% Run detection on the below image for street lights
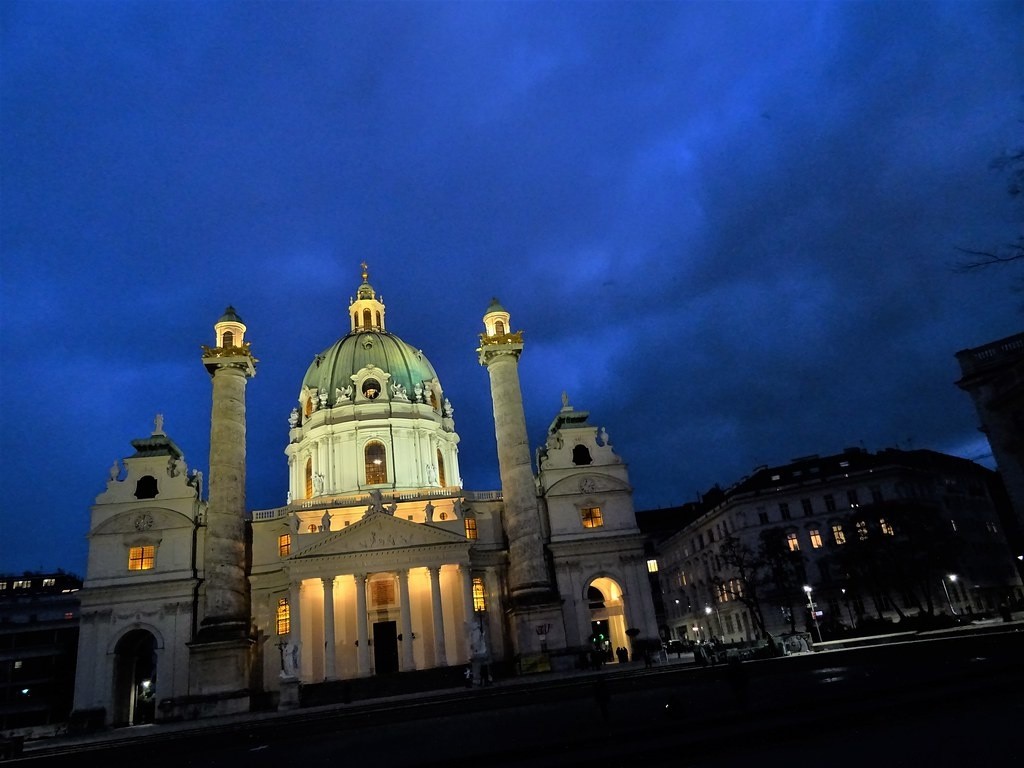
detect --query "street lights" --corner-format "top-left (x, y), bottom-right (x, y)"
top-left (940, 570), bottom-right (957, 615)
top-left (804, 586), bottom-right (822, 641)
top-left (705, 600), bottom-right (725, 644)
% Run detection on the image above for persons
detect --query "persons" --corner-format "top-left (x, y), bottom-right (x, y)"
top-left (471, 621), bottom-right (483, 654)
top-left (590, 646), bottom-right (628, 672)
top-left (322, 510), bottom-right (334, 532)
top-left (369, 488), bottom-right (386, 513)
top-left (290, 510), bottom-right (304, 535)
top-left (280, 632), bottom-right (296, 676)
top-left (453, 496), bottom-right (466, 520)
top-left (422, 501), bottom-right (436, 523)
